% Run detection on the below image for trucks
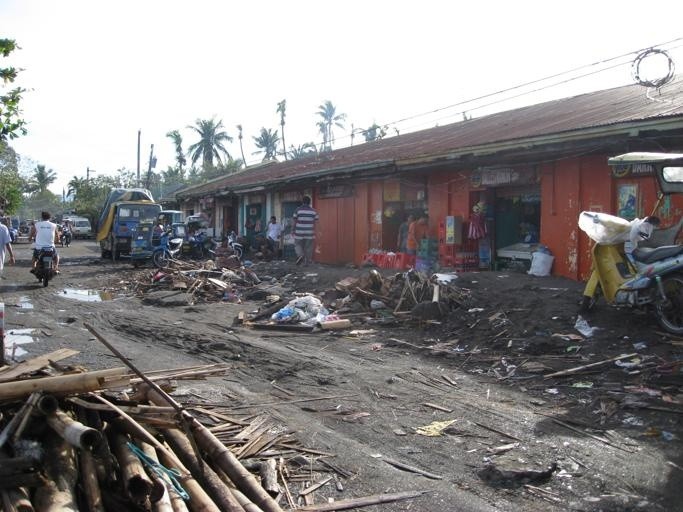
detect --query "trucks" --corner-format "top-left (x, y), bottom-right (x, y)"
top-left (62, 214), bottom-right (91, 239)
top-left (96, 188), bottom-right (162, 261)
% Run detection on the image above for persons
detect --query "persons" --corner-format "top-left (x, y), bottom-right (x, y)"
top-left (29, 211), bottom-right (73, 275)
top-left (291, 196), bottom-right (319, 268)
top-left (0, 216), bottom-right (15, 270)
top-left (397, 212), bottom-right (431, 256)
top-left (266, 216), bottom-right (281, 258)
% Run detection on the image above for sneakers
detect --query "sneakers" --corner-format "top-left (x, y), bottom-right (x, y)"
top-left (295, 256), bottom-right (303, 265)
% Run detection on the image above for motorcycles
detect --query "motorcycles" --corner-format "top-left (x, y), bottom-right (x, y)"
top-left (583, 213), bottom-right (683, 335)
top-left (129, 210), bottom-right (243, 268)
top-left (30, 232), bottom-right (71, 286)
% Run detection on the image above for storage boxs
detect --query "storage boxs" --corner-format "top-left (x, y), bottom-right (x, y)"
top-left (415, 239), bottom-right (438, 274)
